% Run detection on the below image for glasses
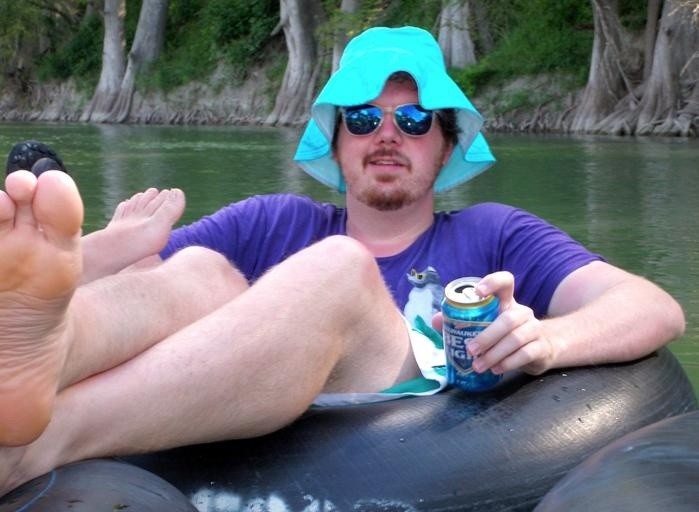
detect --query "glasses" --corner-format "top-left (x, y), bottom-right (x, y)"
top-left (336, 103), bottom-right (444, 137)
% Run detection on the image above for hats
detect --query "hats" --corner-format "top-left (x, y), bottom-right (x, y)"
top-left (293, 26), bottom-right (497, 193)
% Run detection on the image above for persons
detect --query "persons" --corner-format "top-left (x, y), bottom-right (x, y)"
top-left (0, 26), bottom-right (687, 492)
top-left (78, 185), bottom-right (185, 286)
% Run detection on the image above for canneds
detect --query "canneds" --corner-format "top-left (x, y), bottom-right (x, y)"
top-left (442, 277), bottom-right (504, 391)
